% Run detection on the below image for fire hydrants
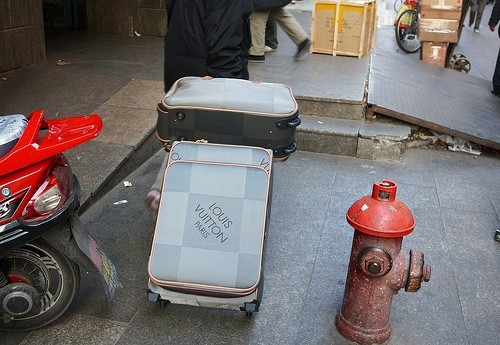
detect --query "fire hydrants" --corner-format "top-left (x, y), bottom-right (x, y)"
top-left (334, 179), bottom-right (433, 345)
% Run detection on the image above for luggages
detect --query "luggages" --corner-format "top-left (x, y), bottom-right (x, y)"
top-left (154, 75), bottom-right (301, 162)
top-left (147, 140), bottom-right (274, 317)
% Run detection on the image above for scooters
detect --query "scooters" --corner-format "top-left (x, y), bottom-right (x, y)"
top-left (0, 108), bottom-right (119, 333)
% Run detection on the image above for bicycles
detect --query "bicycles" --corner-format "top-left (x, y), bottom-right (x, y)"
top-left (394, 0), bottom-right (422, 54)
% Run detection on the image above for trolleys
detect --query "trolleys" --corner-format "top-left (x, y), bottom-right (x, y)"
top-left (419, 0), bottom-right (472, 74)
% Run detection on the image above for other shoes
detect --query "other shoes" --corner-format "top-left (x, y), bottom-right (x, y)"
top-left (474, 30), bottom-right (479, 33)
top-left (468, 23), bottom-right (471, 28)
top-left (264, 45), bottom-right (278, 53)
top-left (295, 39), bottom-right (311, 58)
top-left (248, 54), bottom-right (264, 62)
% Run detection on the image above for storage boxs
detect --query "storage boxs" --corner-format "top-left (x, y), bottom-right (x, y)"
top-left (422, 41), bottom-right (449, 68)
top-left (417, 14), bottom-right (459, 43)
top-left (310, 0), bottom-right (377, 60)
top-left (420, 0), bottom-right (462, 20)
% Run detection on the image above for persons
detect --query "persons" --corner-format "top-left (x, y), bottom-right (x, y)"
top-left (248, 0), bottom-right (312, 63)
top-left (145, 0), bottom-right (252, 220)
top-left (469, 0), bottom-right (489, 34)
top-left (488, 0), bottom-right (500, 96)
